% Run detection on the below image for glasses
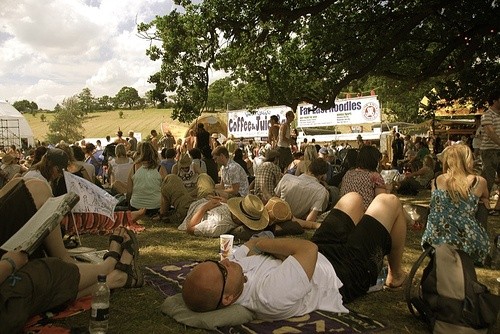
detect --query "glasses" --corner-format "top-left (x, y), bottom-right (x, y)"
top-left (195, 259), bottom-right (228, 310)
top-left (55, 167), bottom-right (63, 173)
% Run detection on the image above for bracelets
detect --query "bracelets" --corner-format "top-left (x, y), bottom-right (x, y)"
top-left (2, 258), bottom-right (16, 277)
top-left (254, 238), bottom-right (265, 253)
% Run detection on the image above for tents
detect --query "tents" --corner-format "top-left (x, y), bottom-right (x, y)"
top-left (0, 99), bottom-right (36, 149)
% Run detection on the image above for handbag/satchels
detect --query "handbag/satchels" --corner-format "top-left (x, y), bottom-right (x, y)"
top-left (229, 225), bottom-right (262, 241)
top-left (272, 221), bottom-right (304, 236)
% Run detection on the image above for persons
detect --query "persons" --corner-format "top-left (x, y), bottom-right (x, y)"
top-left (182, 192), bottom-right (408, 315)
top-left (422, 140), bottom-right (491, 263)
top-left (0, 91), bottom-right (500, 233)
top-left (0, 177), bottom-right (82, 264)
top-left (0, 224), bottom-right (144, 334)
top-left (153, 172), bottom-right (270, 238)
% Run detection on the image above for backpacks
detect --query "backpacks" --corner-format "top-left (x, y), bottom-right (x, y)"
top-left (407, 241), bottom-right (498, 330)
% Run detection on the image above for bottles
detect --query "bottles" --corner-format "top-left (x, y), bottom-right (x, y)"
top-left (88, 274), bottom-right (109, 334)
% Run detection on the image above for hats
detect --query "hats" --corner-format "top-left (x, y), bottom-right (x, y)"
top-left (45, 148), bottom-right (68, 172)
top-left (115, 131), bottom-right (123, 136)
top-left (265, 196), bottom-right (293, 226)
top-left (226, 194), bottom-right (269, 230)
top-left (356, 135), bottom-right (362, 138)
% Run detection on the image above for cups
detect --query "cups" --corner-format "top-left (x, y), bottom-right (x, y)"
top-left (220, 235), bottom-right (235, 260)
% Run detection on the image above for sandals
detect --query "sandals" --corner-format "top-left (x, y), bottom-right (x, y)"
top-left (103, 226), bottom-right (128, 261)
top-left (114, 230), bottom-right (143, 288)
top-left (152, 210), bottom-right (170, 223)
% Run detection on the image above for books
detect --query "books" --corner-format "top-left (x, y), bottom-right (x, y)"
top-left (1, 193), bottom-right (80, 255)
